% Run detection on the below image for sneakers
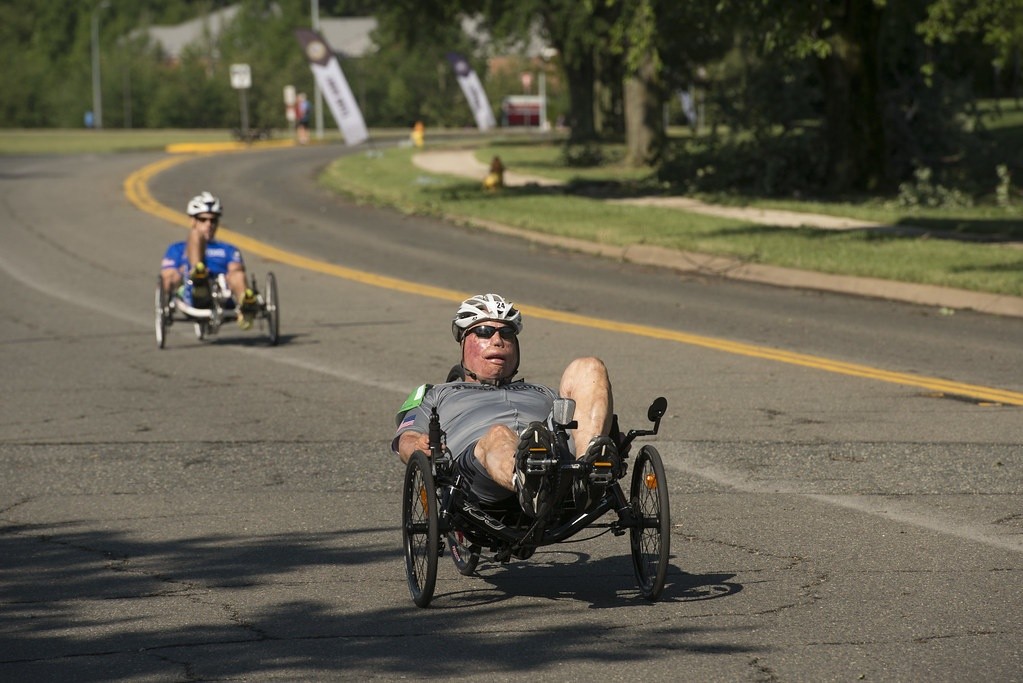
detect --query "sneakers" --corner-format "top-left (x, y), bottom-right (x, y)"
top-left (571, 435), bottom-right (619, 513)
top-left (511, 422), bottom-right (553, 519)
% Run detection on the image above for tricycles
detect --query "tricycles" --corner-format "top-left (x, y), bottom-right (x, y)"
top-left (153, 258), bottom-right (281, 350)
top-left (398, 362), bottom-right (673, 610)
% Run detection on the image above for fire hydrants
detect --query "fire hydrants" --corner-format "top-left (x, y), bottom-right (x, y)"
top-left (481, 154), bottom-right (509, 188)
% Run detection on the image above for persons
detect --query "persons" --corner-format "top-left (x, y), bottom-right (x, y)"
top-left (297, 93), bottom-right (311, 145)
top-left (391, 293), bottom-right (620, 520)
top-left (160, 191), bottom-right (256, 329)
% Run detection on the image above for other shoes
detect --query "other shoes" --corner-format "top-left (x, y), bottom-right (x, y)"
top-left (237, 289), bottom-right (257, 331)
top-left (190, 263), bottom-right (209, 308)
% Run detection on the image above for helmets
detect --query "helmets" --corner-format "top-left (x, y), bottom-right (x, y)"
top-left (187, 191), bottom-right (224, 217)
top-left (451, 293), bottom-right (522, 342)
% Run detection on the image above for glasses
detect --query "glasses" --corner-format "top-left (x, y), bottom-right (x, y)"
top-left (464, 326), bottom-right (517, 340)
top-left (194, 216), bottom-right (218, 224)
